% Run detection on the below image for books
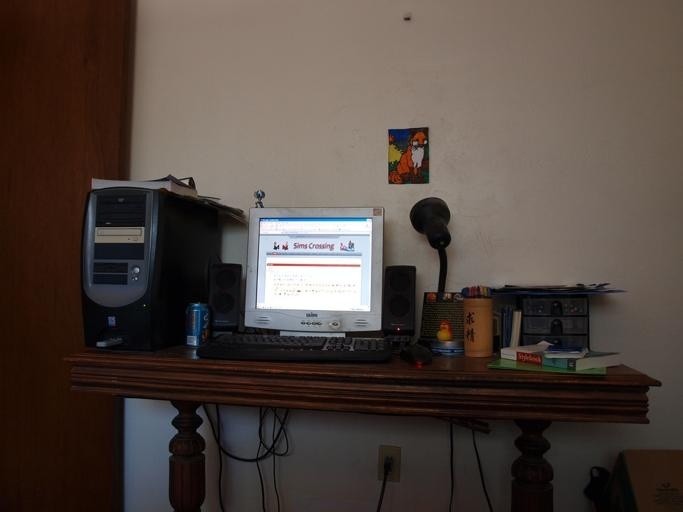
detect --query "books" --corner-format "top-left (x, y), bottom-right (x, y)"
top-left (88, 173), bottom-right (198, 200)
top-left (542, 344), bottom-right (587, 359)
top-left (509, 308), bottom-right (521, 348)
top-left (499, 306), bottom-right (511, 347)
top-left (499, 340), bottom-right (624, 371)
top-left (487, 357), bottom-right (607, 376)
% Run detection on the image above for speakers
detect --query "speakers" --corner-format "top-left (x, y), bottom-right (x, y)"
top-left (206, 262), bottom-right (242, 328)
top-left (385, 264), bottom-right (416, 333)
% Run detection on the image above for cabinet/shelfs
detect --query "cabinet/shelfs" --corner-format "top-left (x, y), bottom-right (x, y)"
top-left (513, 294), bottom-right (590, 352)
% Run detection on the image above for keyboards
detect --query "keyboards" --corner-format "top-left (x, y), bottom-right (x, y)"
top-left (211, 332), bottom-right (391, 364)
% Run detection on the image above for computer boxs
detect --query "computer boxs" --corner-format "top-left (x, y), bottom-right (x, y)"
top-left (80, 189), bottom-right (222, 353)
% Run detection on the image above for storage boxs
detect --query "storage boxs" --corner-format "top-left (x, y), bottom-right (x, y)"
top-left (613, 448), bottom-right (683, 512)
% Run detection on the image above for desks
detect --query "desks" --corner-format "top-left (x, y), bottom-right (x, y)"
top-left (55, 351), bottom-right (663, 512)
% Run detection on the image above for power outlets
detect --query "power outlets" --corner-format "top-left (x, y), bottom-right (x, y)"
top-left (378, 446), bottom-right (402, 480)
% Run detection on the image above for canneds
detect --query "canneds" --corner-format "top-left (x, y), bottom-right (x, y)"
top-left (185, 302), bottom-right (211, 349)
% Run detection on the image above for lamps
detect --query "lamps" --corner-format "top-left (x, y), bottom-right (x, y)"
top-left (410, 195), bottom-right (452, 303)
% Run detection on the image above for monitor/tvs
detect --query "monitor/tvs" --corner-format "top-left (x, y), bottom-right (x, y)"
top-left (243, 206), bottom-right (386, 338)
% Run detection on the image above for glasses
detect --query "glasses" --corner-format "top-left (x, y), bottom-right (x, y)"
top-left (178, 177), bottom-right (197, 190)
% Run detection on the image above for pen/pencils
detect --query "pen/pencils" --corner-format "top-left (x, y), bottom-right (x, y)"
top-left (462, 286), bottom-right (491, 298)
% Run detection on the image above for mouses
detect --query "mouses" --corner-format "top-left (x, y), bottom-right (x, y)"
top-left (403, 343), bottom-right (432, 367)
